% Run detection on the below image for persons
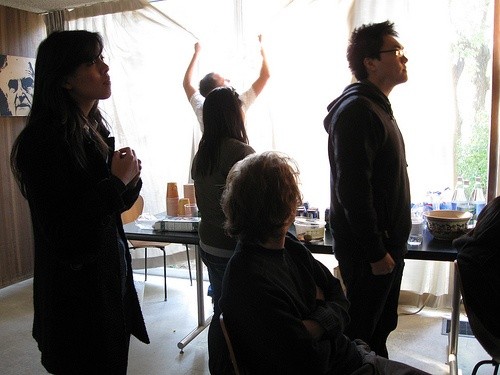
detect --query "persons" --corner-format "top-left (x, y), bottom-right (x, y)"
top-left (218, 152), bottom-right (435, 375)
top-left (182, 34), bottom-right (275, 137)
top-left (192, 86), bottom-right (258, 375)
top-left (324, 21), bottom-right (412, 358)
top-left (453, 195), bottom-right (500, 339)
top-left (0, 55), bottom-right (36, 116)
top-left (11, 30), bottom-right (142, 375)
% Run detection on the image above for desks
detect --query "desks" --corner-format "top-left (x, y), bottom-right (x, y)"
top-left (123, 215), bottom-right (484, 375)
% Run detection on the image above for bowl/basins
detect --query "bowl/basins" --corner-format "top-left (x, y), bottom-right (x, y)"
top-left (423, 210), bottom-right (473, 240)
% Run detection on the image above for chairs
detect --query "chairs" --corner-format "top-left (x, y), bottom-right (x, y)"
top-left (457, 253), bottom-right (500, 375)
top-left (121, 195), bottom-right (192, 301)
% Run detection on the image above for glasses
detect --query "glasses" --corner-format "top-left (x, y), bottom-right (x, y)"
top-left (378, 47), bottom-right (404, 56)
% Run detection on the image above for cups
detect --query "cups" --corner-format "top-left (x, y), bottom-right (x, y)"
top-left (407, 219), bottom-right (424, 245)
top-left (166, 183), bottom-right (198, 220)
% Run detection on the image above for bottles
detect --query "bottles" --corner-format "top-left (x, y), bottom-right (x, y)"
top-left (451, 178), bottom-right (486, 228)
